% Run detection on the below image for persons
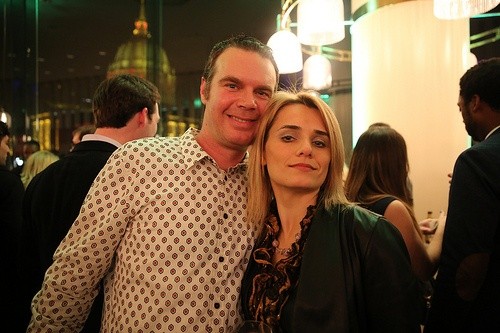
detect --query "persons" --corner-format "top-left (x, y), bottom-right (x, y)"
top-left (422, 60), bottom-right (500, 333)
top-left (235, 77), bottom-right (420, 333)
top-left (343, 122), bottom-right (453, 284)
top-left (24, 74), bottom-right (161, 333)
top-left (0, 119), bottom-right (94, 333)
top-left (26, 33), bottom-right (279, 333)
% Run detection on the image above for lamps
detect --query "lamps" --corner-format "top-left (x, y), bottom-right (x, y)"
top-left (266, 0), bottom-right (345, 91)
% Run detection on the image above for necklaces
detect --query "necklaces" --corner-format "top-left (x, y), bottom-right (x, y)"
top-left (272, 232), bottom-right (301, 257)
